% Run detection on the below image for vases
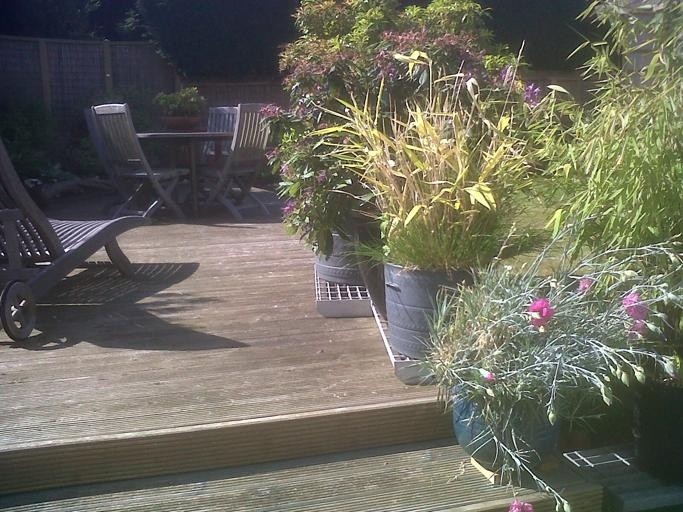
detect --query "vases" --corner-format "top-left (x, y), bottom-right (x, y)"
top-left (446, 346), bottom-right (567, 472)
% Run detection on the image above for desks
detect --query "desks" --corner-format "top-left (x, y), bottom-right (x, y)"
top-left (134, 128), bottom-right (234, 210)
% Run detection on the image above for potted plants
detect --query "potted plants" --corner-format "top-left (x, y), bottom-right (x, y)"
top-left (298, 51), bottom-right (579, 365)
top-left (627, 249), bottom-right (683, 480)
top-left (152, 83), bottom-right (208, 133)
top-left (257, 0), bottom-right (545, 285)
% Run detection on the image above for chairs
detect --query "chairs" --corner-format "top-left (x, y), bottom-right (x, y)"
top-left (0, 126), bottom-right (154, 344)
top-left (89, 100), bottom-right (190, 221)
top-left (188, 98), bottom-right (277, 226)
top-left (196, 100), bottom-right (242, 205)
top-left (78, 104), bottom-right (163, 209)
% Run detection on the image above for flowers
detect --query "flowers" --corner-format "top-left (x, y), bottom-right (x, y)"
top-left (402, 215), bottom-right (683, 511)
